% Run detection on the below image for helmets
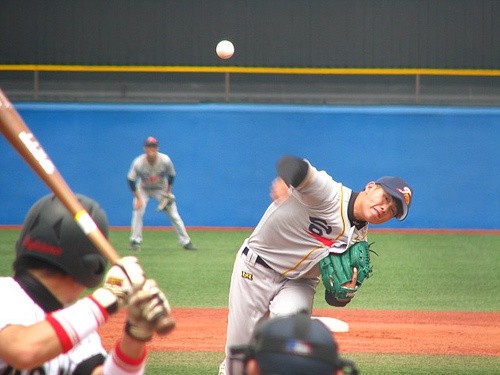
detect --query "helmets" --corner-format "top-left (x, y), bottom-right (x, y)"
top-left (13, 191), bottom-right (109, 288)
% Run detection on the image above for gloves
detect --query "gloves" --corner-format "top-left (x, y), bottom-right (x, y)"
top-left (91, 256), bottom-right (145, 317)
top-left (125, 279), bottom-right (170, 341)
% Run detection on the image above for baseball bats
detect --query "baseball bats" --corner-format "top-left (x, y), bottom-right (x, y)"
top-left (0, 87), bottom-right (175, 335)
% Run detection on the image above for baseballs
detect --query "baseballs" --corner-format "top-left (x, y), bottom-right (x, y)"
top-left (216, 39), bottom-right (235, 60)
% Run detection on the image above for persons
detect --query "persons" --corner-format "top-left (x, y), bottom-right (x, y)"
top-left (127, 137), bottom-right (197, 249)
top-left (229, 313), bottom-right (358, 375)
top-left (217, 152), bottom-right (413, 375)
top-left (0, 188), bottom-right (170, 375)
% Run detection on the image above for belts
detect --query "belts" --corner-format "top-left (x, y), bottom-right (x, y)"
top-left (243, 247), bottom-right (272, 269)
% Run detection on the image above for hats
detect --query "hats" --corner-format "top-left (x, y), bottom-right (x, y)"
top-left (144, 136), bottom-right (158, 146)
top-left (375, 176), bottom-right (414, 221)
top-left (243, 309), bottom-right (337, 366)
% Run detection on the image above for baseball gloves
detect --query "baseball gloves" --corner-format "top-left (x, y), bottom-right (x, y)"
top-left (319, 241), bottom-right (371, 298)
top-left (157, 194), bottom-right (175, 211)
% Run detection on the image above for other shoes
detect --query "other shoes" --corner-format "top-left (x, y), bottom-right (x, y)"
top-left (129, 243), bottom-right (140, 250)
top-left (184, 242), bottom-right (198, 250)
